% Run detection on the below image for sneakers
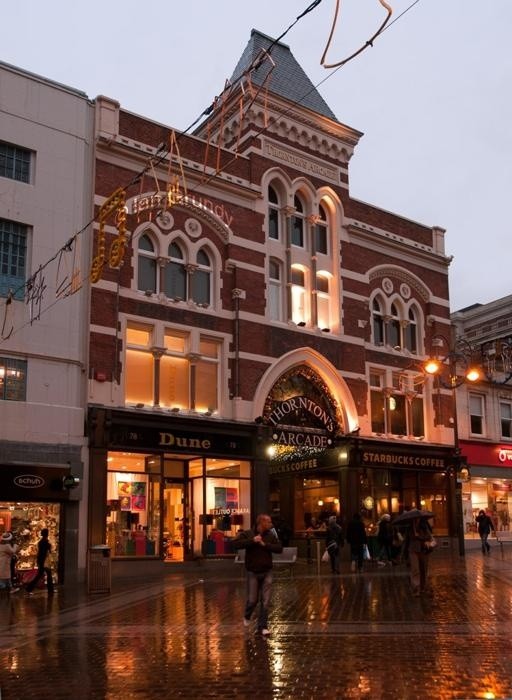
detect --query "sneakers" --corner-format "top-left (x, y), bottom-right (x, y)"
top-left (260, 628), bottom-right (270, 635)
top-left (243, 616), bottom-right (250, 627)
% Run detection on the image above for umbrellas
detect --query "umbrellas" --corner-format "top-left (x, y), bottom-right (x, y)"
top-left (392, 507), bottom-right (436, 525)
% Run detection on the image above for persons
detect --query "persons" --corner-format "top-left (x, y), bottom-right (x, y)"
top-left (474, 509), bottom-right (495, 554)
top-left (119, 496), bottom-right (129, 508)
top-left (0, 528), bottom-right (22, 594)
top-left (23, 528), bottom-right (59, 594)
top-left (226, 514), bottom-right (282, 637)
top-left (324, 510), bottom-right (437, 597)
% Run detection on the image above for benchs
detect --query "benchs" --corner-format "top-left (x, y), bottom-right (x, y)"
top-left (234, 547), bottom-right (297, 582)
top-left (492, 530), bottom-right (512, 552)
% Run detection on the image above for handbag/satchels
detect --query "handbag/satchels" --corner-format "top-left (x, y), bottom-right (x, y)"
top-left (422, 540), bottom-right (436, 555)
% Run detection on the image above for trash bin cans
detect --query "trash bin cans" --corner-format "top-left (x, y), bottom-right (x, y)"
top-left (87, 546), bottom-right (113, 596)
